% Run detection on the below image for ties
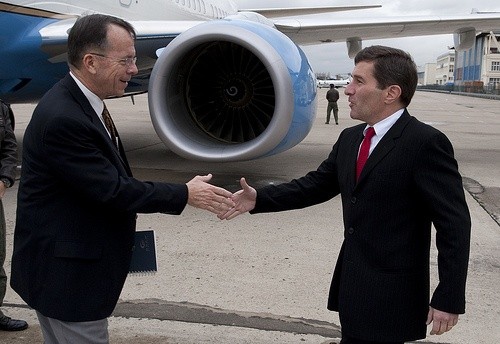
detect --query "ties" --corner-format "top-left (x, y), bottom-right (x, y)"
top-left (356, 126), bottom-right (376, 184)
top-left (103, 103), bottom-right (119, 155)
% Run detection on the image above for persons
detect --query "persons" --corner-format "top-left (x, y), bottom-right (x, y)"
top-left (0, 99), bottom-right (28, 331)
top-left (217, 45), bottom-right (472, 344)
top-left (9, 14), bottom-right (236, 344)
top-left (325, 83), bottom-right (339, 125)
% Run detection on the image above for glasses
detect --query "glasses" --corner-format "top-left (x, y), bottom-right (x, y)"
top-left (83, 51), bottom-right (137, 69)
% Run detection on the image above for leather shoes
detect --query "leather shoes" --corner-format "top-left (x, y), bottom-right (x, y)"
top-left (0, 315), bottom-right (29, 331)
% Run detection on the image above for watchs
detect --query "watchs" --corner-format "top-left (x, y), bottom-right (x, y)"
top-left (0, 177), bottom-right (11, 188)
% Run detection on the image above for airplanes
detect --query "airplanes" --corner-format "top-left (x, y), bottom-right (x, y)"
top-left (0, 0), bottom-right (500, 163)
top-left (317, 79), bottom-right (350, 89)
